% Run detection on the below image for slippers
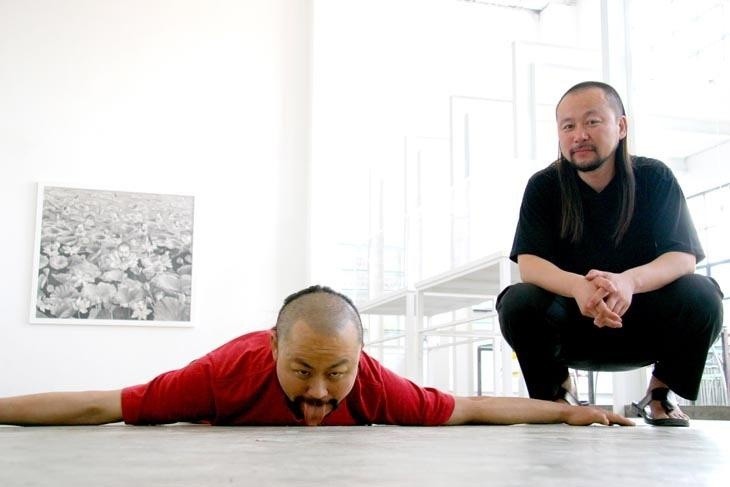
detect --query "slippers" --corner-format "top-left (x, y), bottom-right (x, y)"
top-left (630, 387), bottom-right (691, 426)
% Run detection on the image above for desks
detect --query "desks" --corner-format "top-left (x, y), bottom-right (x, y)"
top-left (352, 242), bottom-right (521, 399)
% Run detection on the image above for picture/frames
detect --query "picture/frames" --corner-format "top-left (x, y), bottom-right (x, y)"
top-left (29, 183), bottom-right (199, 329)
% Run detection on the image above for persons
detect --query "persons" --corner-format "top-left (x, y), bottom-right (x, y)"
top-left (495, 78), bottom-right (726, 426)
top-left (1, 285), bottom-right (635, 427)
